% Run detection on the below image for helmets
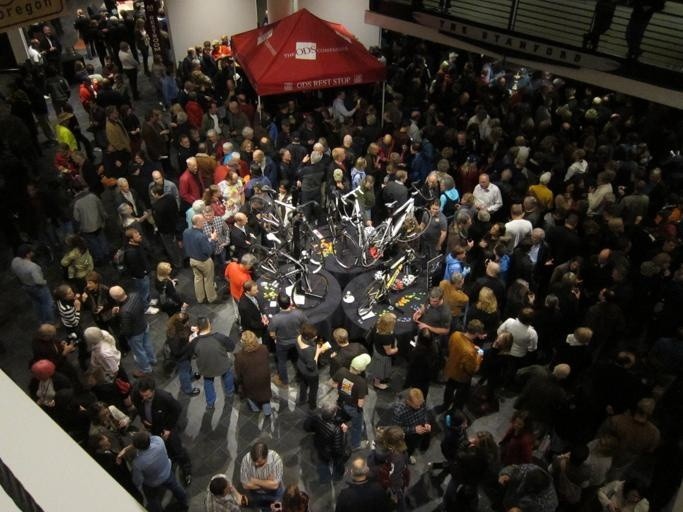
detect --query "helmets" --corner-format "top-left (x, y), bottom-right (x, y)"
top-left (510, 203), bottom-right (526, 220)
top-left (522, 196), bottom-right (537, 213)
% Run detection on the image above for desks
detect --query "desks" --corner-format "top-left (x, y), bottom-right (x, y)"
top-left (343, 270), bottom-right (434, 359)
top-left (250, 263), bottom-right (342, 352)
top-left (308, 220), bottom-right (382, 285)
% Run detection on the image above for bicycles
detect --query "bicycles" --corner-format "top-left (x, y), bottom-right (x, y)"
top-left (330, 185), bottom-right (368, 269)
top-left (246, 231), bottom-right (329, 310)
top-left (357, 244), bottom-right (427, 318)
top-left (254, 197), bottom-right (324, 270)
top-left (362, 179), bottom-right (436, 268)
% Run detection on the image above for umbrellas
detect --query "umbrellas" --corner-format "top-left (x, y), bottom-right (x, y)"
top-left (230, 8), bottom-right (386, 125)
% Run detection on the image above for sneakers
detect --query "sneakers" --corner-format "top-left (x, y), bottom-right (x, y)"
top-left (150, 297), bottom-right (160, 305)
top-left (410, 456), bottom-right (419, 464)
top-left (272, 374), bottom-right (291, 389)
top-left (145, 306), bottom-right (160, 314)
top-left (189, 386), bottom-right (202, 396)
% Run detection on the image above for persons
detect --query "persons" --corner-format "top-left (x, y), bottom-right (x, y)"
top-left (126, 433), bottom-right (189, 511)
top-left (120, 102), bottom-right (141, 150)
top-left (499, 465), bottom-right (557, 511)
top-left (499, 206), bottom-right (532, 255)
top-left (205, 475), bottom-right (247, 511)
top-left (238, 279), bottom-right (275, 354)
top-left (506, 280), bottom-right (535, 318)
top-left (182, 215), bottom-right (218, 303)
top-left (473, 262), bottom-right (504, 308)
top-left (149, 170), bottom-right (180, 212)
top-left (135, 150), bottom-right (157, 182)
top-left (528, 169), bottom-right (552, 206)
top-left (513, 365), bottom-right (570, 430)
top-left (84, 64), bottom-right (103, 83)
top-left (499, 412), bottom-right (532, 465)
top-left (110, 286), bottom-right (158, 372)
top-left (386, 45), bottom-right (410, 123)
top-left (196, 143), bottom-right (215, 187)
top-left (552, 72), bottom-right (681, 287)
top-left (467, 212), bottom-right (494, 260)
top-left (71, 148), bottom-right (104, 199)
top-left (420, 201), bottom-right (447, 266)
top-left (151, 184), bottom-right (182, 279)
top-left (61, 235), bottom-right (95, 292)
top-left (466, 287), bottom-right (502, 341)
top-left (530, 419), bottom-right (563, 466)
top-left (74, 10), bottom-right (97, 60)
top-left (269, 292), bottom-right (310, 388)
top-left (598, 473), bottom-right (649, 511)
top-left (538, 276), bottom-right (600, 363)
top-left (143, 109), bottom-right (169, 161)
top-left (127, 163), bottom-right (151, 210)
top-left (480, 156), bottom-right (551, 169)
top-left (433, 320), bottom-right (483, 417)
top-left (133, 2), bottom-right (145, 20)
top-left (477, 334), bottom-right (513, 387)
top-left (96, 78), bottom-right (120, 107)
top-left (75, 61), bottom-right (89, 85)
top-left (220, 142), bottom-right (234, 164)
top-left (41, 27), bottom-right (62, 75)
top-left (225, 254), bottom-right (256, 332)
top-left (217, 169), bottom-right (245, 218)
top-left (244, 93), bottom-right (411, 221)
top-left (328, 354), bottom-right (371, 451)
top-left (29, 360), bottom-right (87, 446)
top-left (496, 308), bottom-right (538, 395)
top-left (33, 322), bottom-right (77, 387)
top-left (106, 107), bottom-right (132, 153)
top-left (509, 239), bottom-right (535, 291)
top-left (230, 151), bottom-right (247, 176)
top-left (162, 312), bottom-right (200, 397)
top-left (205, 185), bottom-right (225, 217)
top-left (168, 34), bottom-right (264, 141)
top-left (403, 328), bottom-right (440, 403)
top-left (548, 445), bottom-right (589, 510)
top-left (439, 273), bottom-right (468, 330)
top-left (4, 87), bottom-right (49, 252)
top-left (124, 228), bottom-right (159, 316)
top-left (335, 459), bottom-right (395, 511)
top-left (411, 38), bottom-right (546, 157)
top-left (54, 103), bottom-right (82, 152)
top-left (90, 401), bottom-right (132, 452)
top-left (120, 10), bottom-right (143, 65)
top-left (118, 203), bottom-right (151, 248)
top-left (88, 434), bottom-right (143, 504)
top-left (134, 19), bottom-right (150, 75)
top-left (459, 432), bottom-right (496, 510)
top-left (179, 157), bottom-right (204, 229)
top-left (369, 427), bottom-right (409, 503)
top-left (296, 322), bottom-right (320, 414)
top-left (598, 289), bottom-right (682, 472)
top-left (443, 247), bottom-right (470, 283)
top-left (88, 19), bottom-right (108, 69)
top-left (130, 379), bottom-right (191, 482)
top-left (82, 270), bottom-right (115, 329)
top-left (46, 65), bottom-right (71, 116)
top-left (234, 331), bottom-right (272, 417)
top-left (413, 287), bottom-right (451, 385)
top-left (115, 178), bottom-right (145, 216)
top-left (437, 176), bottom-right (461, 215)
top-left (54, 285), bottom-right (81, 336)
top-left (176, 136), bottom-right (196, 175)
top-left (73, 184), bottom-right (109, 259)
top-left (103, 16), bottom-right (129, 74)
top-left (366, 312), bottom-right (398, 389)
top-left (496, 169), bottom-right (515, 208)
top-left (527, 229), bottom-right (549, 275)
top-left (186, 200), bottom-right (204, 224)
top-left (422, 159), bottom-right (457, 205)
top-left (27, 39), bottom-right (50, 99)
top-left (424, 411), bottom-right (471, 498)
top-left (80, 76), bottom-right (96, 113)
top-left (448, 212), bottom-right (472, 250)
top-left (187, 316), bottom-right (236, 411)
top-left (524, 198), bottom-right (543, 229)
top-left (304, 404), bottom-right (348, 481)
top-left (12, 244), bottom-right (56, 322)
top-left (472, 174), bottom-right (502, 211)
top-left (84, 327), bottom-right (130, 399)
top-left (241, 443), bottom-right (284, 511)
top-left (330, 329), bottom-right (368, 395)
top-left (231, 212), bottom-right (255, 264)
top-left (53, 143), bottom-right (75, 178)
top-left (202, 206), bottom-right (230, 281)
top-left (154, 262), bottom-right (187, 319)
top-left (395, 389), bottom-right (431, 463)
top-left (459, 158), bottom-right (480, 194)
top-left (118, 43), bottom-right (143, 100)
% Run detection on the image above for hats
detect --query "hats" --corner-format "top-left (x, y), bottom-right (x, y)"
top-left (56, 111), bottom-right (75, 126)
top-left (349, 352), bottom-right (372, 371)
top-left (381, 423), bottom-right (409, 453)
top-left (631, 396), bottom-right (657, 423)
top-left (539, 171), bottom-right (552, 185)
top-left (448, 410), bottom-right (466, 426)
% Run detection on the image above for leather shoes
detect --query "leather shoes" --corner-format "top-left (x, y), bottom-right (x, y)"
top-left (180, 467), bottom-right (193, 487)
top-left (132, 369), bottom-right (155, 378)
top-left (372, 382), bottom-right (392, 391)
